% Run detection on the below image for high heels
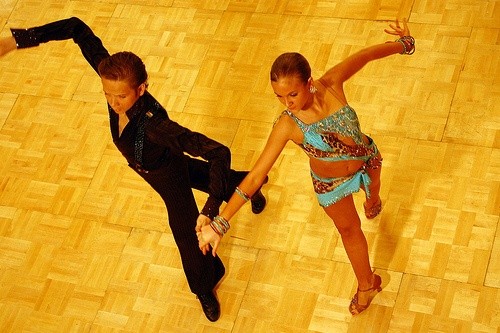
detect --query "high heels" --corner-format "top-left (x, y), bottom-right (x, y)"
top-left (363, 197), bottom-right (381, 219)
top-left (348, 270), bottom-right (381, 316)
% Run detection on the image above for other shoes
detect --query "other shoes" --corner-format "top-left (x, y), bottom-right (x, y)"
top-left (198, 292), bottom-right (220, 322)
top-left (251, 175), bottom-right (269, 214)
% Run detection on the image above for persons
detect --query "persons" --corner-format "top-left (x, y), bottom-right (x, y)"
top-left (1, 15), bottom-right (268, 323)
top-left (195, 19), bottom-right (415, 315)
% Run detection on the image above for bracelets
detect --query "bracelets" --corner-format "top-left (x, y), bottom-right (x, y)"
top-left (392, 35), bottom-right (415, 56)
top-left (235, 187), bottom-right (250, 203)
top-left (209, 214), bottom-right (230, 238)
